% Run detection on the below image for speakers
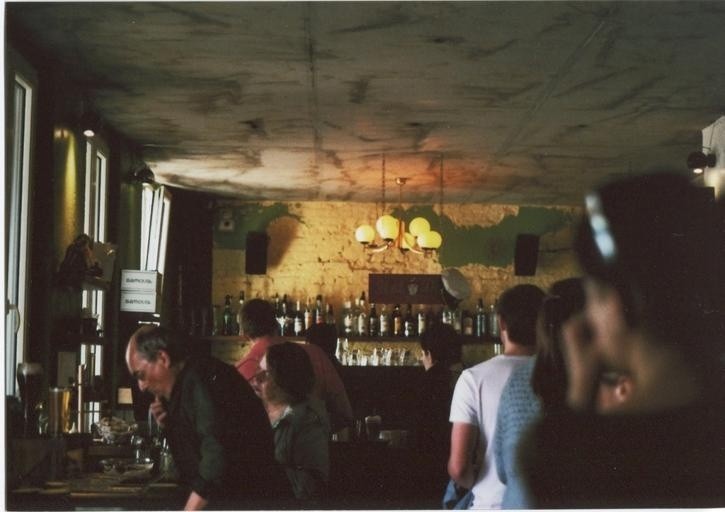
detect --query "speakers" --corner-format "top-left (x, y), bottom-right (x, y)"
top-left (245, 231), bottom-right (268, 274)
top-left (514, 234), bottom-right (539, 276)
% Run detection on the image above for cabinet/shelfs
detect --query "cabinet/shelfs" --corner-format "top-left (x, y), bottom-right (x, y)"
top-left (205, 333), bottom-right (504, 392)
top-left (53, 274), bottom-right (113, 401)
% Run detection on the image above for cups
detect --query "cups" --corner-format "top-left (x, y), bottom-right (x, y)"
top-left (342, 346), bottom-right (406, 367)
top-left (335, 337), bottom-right (348, 359)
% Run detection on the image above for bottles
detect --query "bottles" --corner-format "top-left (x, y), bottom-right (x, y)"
top-left (272, 291), bottom-right (336, 338)
top-left (235, 290), bottom-right (246, 335)
top-left (160, 436), bottom-right (174, 472)
top-left (341, 290), bottom-right (500, 339)
top-left (223, 295), bottom-right (233, 335)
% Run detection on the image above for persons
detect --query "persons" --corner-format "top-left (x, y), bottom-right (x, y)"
top-left (249, 341), bottom-right (333, 511)
top-left (379, 322), bottom-right (467, 429)
top-left (231, 297), bottom-right (355, 438)
top-left (488, 276), bottom-right (588, 508)
top-left (444, 282), bottom-right (546, 509)
top-left (124, 324), bottom-right (299, 511)
top-left (508, 165), bottom-right (725, 509)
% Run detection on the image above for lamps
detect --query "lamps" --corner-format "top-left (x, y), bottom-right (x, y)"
top-left (355, 177), bottom-right (442, 258)
